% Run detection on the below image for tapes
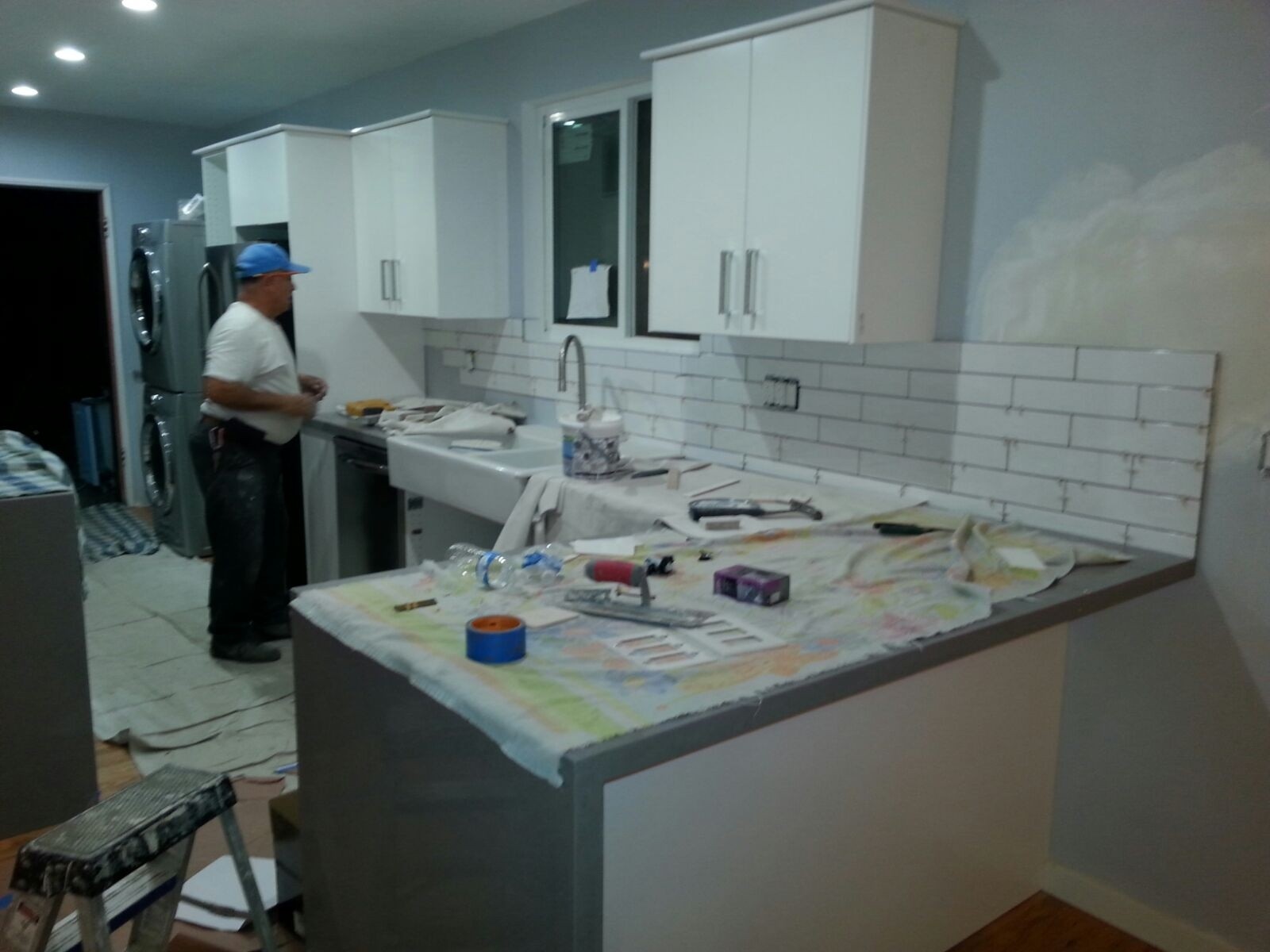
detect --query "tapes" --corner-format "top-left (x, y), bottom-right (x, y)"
top-left (465, 615), bottom-right (525, 663)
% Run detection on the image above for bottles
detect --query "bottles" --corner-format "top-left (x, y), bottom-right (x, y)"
top-left (522, 539), bottom-right (566, 586)
top-left (446, 543), bottom-right (538, 597)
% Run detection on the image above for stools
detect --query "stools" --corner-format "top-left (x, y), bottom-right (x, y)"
top-left (13, 765), bottom-right (276, 952)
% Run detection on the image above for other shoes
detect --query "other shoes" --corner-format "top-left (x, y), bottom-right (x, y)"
top-left (208, 641), bottom-right (280, 664)
top-left (251, 621), bottom-right (292, 638)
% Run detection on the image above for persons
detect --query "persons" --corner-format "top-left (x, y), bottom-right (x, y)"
top-left (188, 241), bottom-right (329, 663)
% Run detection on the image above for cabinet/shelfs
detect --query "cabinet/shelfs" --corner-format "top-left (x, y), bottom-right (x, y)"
top-left (346, 103), bottom-right (524, 321)
top-left (0, 429), bottom-right (97, 846)
top-left (640, 1), bottom-right (969, 350)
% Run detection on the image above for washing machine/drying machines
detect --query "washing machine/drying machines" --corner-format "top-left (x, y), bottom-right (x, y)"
top-left (126, 219), bottom-right (216, 561)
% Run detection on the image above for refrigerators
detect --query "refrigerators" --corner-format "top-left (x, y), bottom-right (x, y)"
top-left (195, 242), bottom-right (295, 363)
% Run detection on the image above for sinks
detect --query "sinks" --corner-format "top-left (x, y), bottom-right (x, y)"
top-left (386, 426), bottom-right (557, 506)
top-left (468, 447), bottom-right (571, 528)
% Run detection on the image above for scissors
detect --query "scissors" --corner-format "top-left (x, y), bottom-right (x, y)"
top-left (872, 521), bottom-right (942, 535)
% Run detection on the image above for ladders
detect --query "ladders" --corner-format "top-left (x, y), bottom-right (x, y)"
top-left (10, 765), bottom-right (279, 951)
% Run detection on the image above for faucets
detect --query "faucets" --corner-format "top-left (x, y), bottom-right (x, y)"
top-left (557, 333), bottom-right (588, 464)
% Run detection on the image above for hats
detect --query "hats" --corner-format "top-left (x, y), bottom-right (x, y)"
top-left (233, 241), bottom-right (311, 279)
